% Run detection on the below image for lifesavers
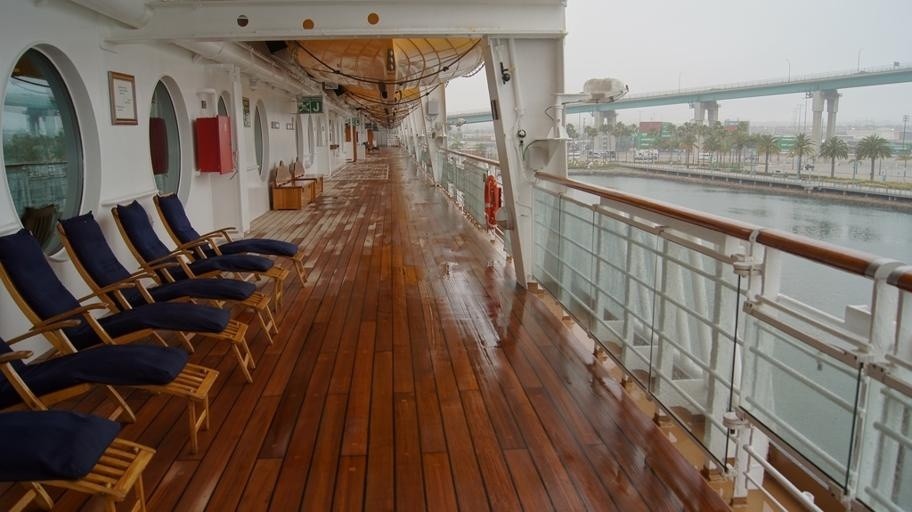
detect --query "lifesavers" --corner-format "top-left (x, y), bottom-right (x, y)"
top-left (484, 175), bottom-right (499, 229)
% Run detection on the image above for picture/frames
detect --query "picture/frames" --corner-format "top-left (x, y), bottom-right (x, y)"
top-left (241, 95), bottom-right (251, 127)
top-left (107, 69), bottom-right (140, 126)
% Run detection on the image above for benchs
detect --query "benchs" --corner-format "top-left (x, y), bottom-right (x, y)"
top-left (271, 157), bottom-right (325, 209)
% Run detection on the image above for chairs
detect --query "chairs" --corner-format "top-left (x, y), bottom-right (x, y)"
top-left (1, 225), bottom-right (258, 384)
top-left (110, 200), bottom-right (291, 314)
top-left (2, 409), bottom-right (158, 512)
top-left (1, 318), bottom-right (223, 459)
top-left (153, 191), bottom-right (309, 289)
top-left (55, 210), bottom-right (278, 345)
